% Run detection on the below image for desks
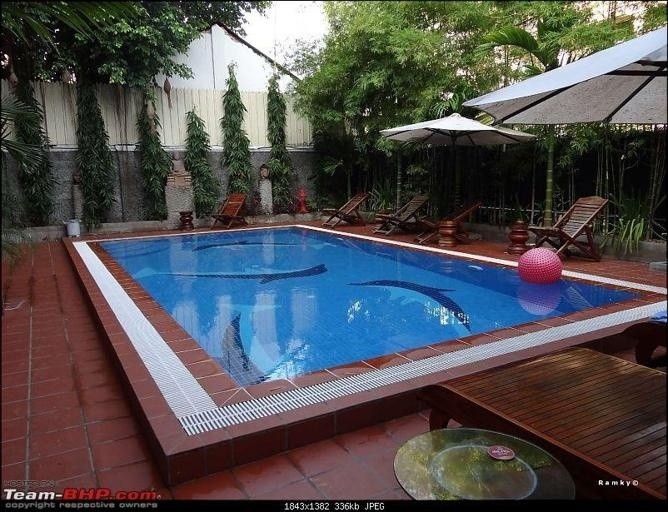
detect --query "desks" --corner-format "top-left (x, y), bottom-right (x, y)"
top-left (393, 428), bottom-right (577, 500)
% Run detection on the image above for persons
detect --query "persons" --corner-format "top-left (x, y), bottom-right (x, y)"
top-left (460, 24), bottom-right (668, 125)
top-left (379, 113), bottom-right (536, 223)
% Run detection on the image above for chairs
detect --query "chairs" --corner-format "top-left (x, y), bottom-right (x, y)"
top-left (373, 194), bottom-right (430, 235)
top-left (417, 321), bottom-right (668, 512)
top-left (415, 199), bottom-right (483, 244)
top-left (321, 192), bottom-right (370, 228)
top-left (211, 192), bottom-right (248, 228)
top-left (528, 196), bottom-right (609, 263)
